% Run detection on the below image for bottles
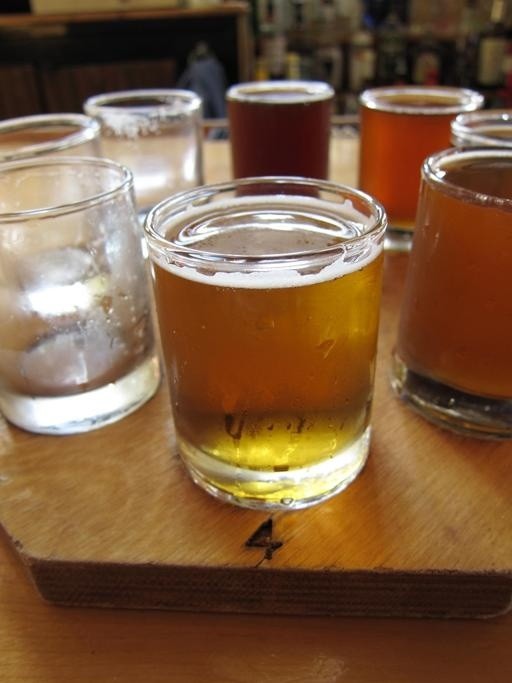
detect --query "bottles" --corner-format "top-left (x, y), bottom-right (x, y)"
top-left (252, 0), bottom-right (512, 94)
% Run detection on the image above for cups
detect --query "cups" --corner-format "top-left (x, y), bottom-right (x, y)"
top-left (0, 154), bottom-right (164, 435)
top-left (226, 78), bottom-right (334, 198)
top-left (0, 112), bottom-right (112, 320)
top-left (144, 173), bottom-right (391, 513)
top-left (388, 142), bottom-right (512, 441)
top-left (356, 85), bottom-right (484, 251)
top-left (81, 88), bottom-right (204, 225)
